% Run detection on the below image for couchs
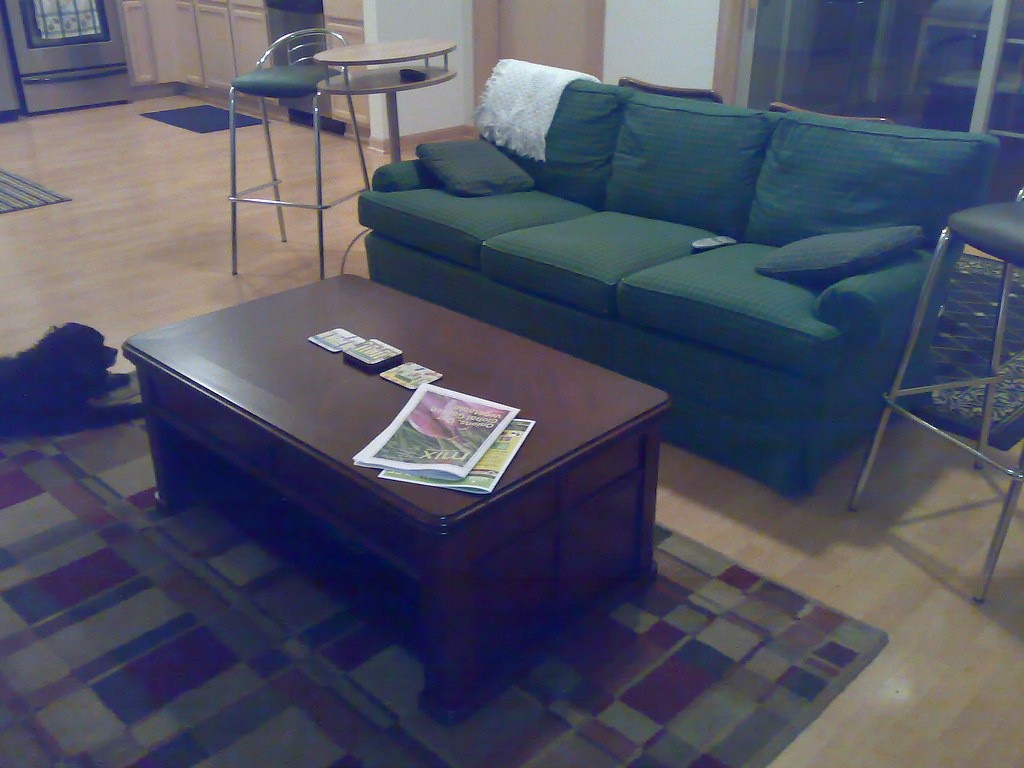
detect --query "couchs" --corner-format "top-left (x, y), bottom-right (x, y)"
top-left (356, 54), bottom-right (1000, 507)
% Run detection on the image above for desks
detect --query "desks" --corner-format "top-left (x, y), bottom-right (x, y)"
top-left (314, 41), bottom-right (460, 276)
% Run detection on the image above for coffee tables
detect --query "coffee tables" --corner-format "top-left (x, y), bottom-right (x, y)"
top-left (122, 273), bottom-right (677, 727)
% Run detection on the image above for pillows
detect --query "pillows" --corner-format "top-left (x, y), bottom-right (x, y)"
top-left (415, 139), bottom-right (535, 198)
top-left (751, 224), bottom-right (927, 290)
top-left (476, 59), bottom-right (634, 213)
top-left (751, 110), bottom-right (1002, 255)
top-left (609, 91), bottom-right (783, 243)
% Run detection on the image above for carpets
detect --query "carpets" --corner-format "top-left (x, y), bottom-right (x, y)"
top-left (0, 168), bottom-right (72, 213)
top-left (138, 104), bottom-right (270, 134)
top-left (0, 368), bottom-right (889, 768)
top-left (907, 254), bottom-right (1024, 451)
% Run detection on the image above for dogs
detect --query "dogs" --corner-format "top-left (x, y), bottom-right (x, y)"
top-left (0, 322), bottom-right (145, 437)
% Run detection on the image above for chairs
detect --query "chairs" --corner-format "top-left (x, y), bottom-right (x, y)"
top-left (227, 26), bottom-right (373, 283)
top-left (847, 200), bottom-right (1024, 606)
